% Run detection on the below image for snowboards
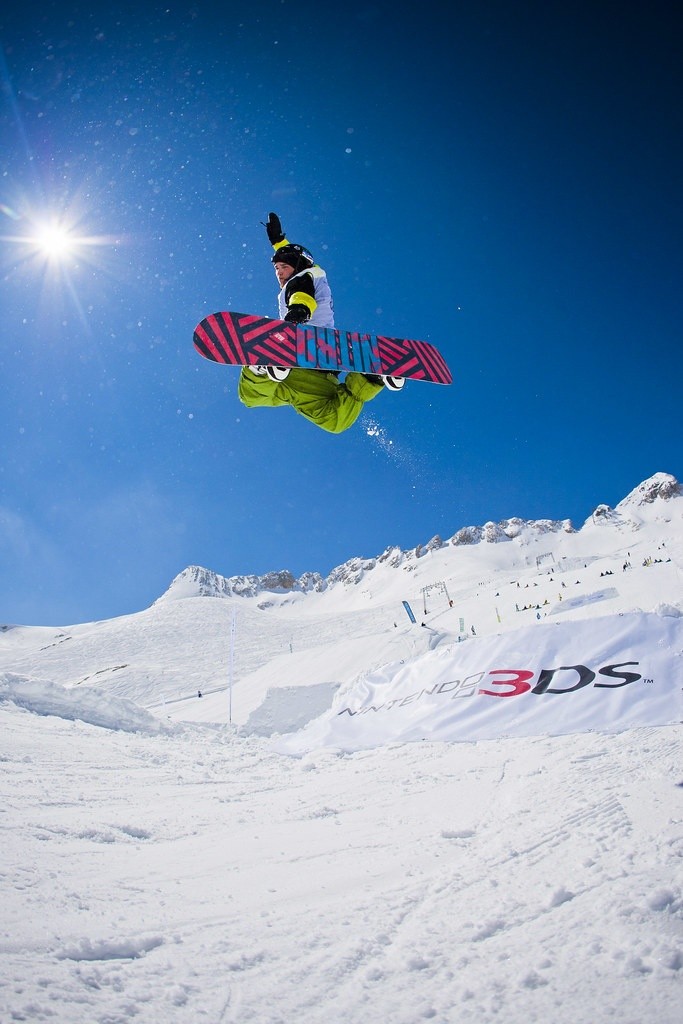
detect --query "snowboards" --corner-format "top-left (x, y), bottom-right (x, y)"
top-left (193, 310), bottom-right (453, 386)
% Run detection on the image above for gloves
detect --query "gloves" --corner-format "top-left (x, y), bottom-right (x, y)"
top-left (265, 212), bottom-right (286, 244)
top-left (284, 308), bottom-right (310, 325)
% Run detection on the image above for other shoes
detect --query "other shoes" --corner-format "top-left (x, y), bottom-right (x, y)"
top-left (379, 375), bottom-right (406, 392)
top-left (265, 365), bottom-right (291, 382)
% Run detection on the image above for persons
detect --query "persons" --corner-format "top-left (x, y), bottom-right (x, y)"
top-left (238, 212), bottom-right (404, 433)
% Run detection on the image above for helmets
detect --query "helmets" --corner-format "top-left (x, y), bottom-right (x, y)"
top-left (270, 244), bottom-right (315, 271)
